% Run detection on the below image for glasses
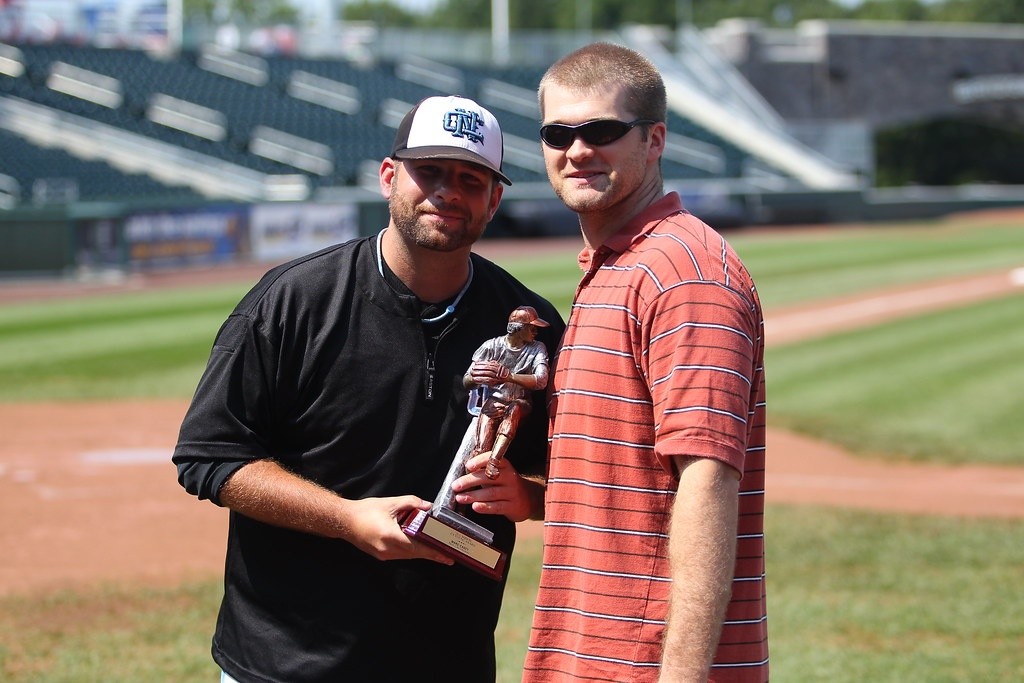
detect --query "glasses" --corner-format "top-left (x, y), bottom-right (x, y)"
top-left (540, 118), bottom-right (655, 150)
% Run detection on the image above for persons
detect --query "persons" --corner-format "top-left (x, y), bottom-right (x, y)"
top-left (463, 305), bottom-right (549, 479)
top-left (172, 94), bottom-right (568, 683)
top-left (536, 43), bottom-right (771, 683)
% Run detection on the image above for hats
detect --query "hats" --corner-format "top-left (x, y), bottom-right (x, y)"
top-left (388, 94), bottom-right (513, 187)
